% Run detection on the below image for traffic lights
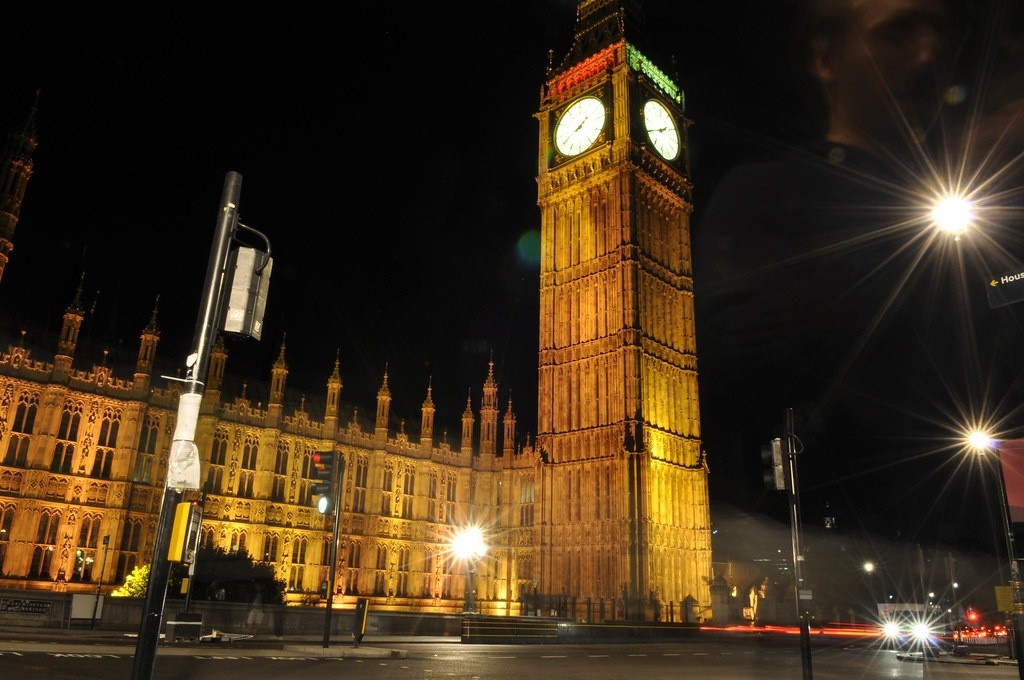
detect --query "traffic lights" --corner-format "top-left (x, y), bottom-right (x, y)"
top-left (970, 612), bottom-right (978, 621)
top-left (311, 451), bottom-right (335, 513)
top-left (761, 439), bottom-right (785, 491)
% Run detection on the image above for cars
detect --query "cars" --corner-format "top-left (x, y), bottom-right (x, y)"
top-left (882, 611), bottom-right (946, 648)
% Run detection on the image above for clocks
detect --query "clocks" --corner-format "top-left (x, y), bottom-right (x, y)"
top-left (639, 98), bottom-right (682, 163)
top-left (553, 95), bottom-right (608, 159)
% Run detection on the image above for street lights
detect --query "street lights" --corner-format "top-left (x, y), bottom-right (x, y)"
top-left (864, 563), bottom-right (875, 622)
top-left (965, 429), bottom-right (1024, 680)
top-left (450, 525), bottom-right (486, 611)
